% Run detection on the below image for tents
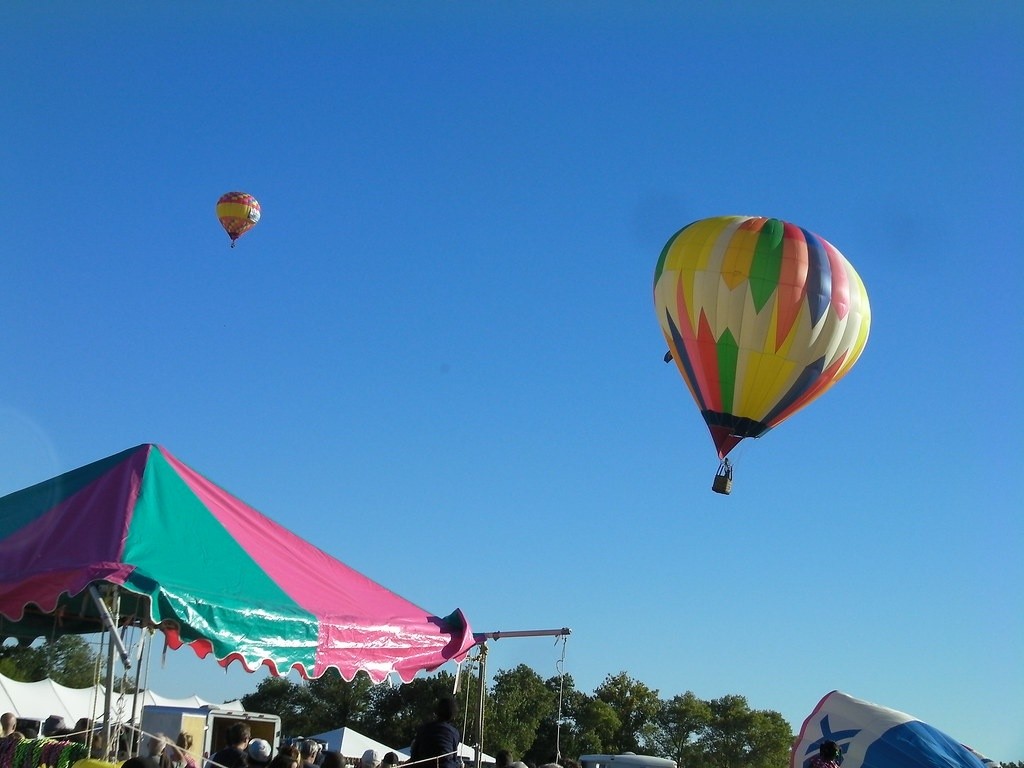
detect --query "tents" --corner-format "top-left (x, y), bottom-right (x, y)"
top-left (0, 440), bottom-right (476, 768)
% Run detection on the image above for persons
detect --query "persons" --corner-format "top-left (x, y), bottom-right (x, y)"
top-left (724, 468), bottom-right (730, 478)
top-left (1, 697), bottom-right (564, 768)
top-left (807, 738), bottom-right (840, 768)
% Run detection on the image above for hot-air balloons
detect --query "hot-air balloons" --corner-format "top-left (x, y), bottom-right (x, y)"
top-left (653, 215), bottom-right (872, 495)
top-left (216, 191), bottom-right (261, 249)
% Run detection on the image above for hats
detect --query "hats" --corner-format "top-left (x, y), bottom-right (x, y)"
top-left (41, 715), bottom-right (66, 735)
top-left (361, 749), bottom-right (381, 768)
top-left (382, 752), bottom-right (398, 764)
top-left (248, 738), bottom-right (271, 762)
top-left (434, 698), bottom-right (460, 715)
